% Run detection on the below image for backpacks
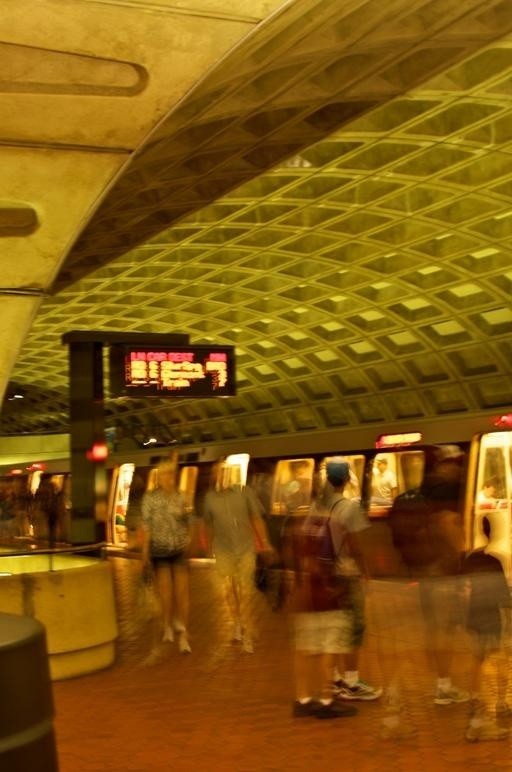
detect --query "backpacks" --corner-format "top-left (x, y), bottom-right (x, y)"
top-left (389, 489), bottom-right (447, 565)
top-left (307, 523), bottom-right (336, 574)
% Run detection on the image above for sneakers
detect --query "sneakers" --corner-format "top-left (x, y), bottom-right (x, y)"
top-left (162, 627), bottom-right (175, 643)
top-left (337, 680), bottom-right (383, 701)
top-left (177, 640), bottom-right (192, 653)
top-left (171, 621), bottom-right (185, 632)
top-left (464, 723), bottom-right (512, 742)
top-left (231, 626), bottom-right (242, 641)
top-left (433, 686), bottom-right (470, 705)
top-left (324, 679), bottom-right (343, 695)
top-left (240, 638), bottom-right (254, 654)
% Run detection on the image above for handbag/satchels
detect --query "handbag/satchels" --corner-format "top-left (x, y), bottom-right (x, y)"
top-left (252, 525), bottom-right (274, 557)
top-left (133, 570), bottom-right (162, 619)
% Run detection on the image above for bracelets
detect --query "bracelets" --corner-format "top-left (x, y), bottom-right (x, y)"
top-left (261, 537), bottom-right (271, 544)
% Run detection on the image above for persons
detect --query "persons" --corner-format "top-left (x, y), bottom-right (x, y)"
top-left (139, 460), bottom-right (198, 656)
top-left (281, 443), bottom-right (512, 745)
top-left (0, 471), bottom-right (60, 553)
top-left (202, 458), bottom-right (275, 657)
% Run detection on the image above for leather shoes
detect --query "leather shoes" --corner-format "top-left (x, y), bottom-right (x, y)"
top-left (292, 700), bottom-right (315, 716)
top-left (314, 701), bottom-right (357, 720)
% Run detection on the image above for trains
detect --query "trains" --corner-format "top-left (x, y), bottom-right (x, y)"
top-left (0, 413), bottom-right (512, 581)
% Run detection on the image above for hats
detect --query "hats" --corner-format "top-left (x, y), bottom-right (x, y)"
top-left (326, 460), bottom-right (350, 487)
